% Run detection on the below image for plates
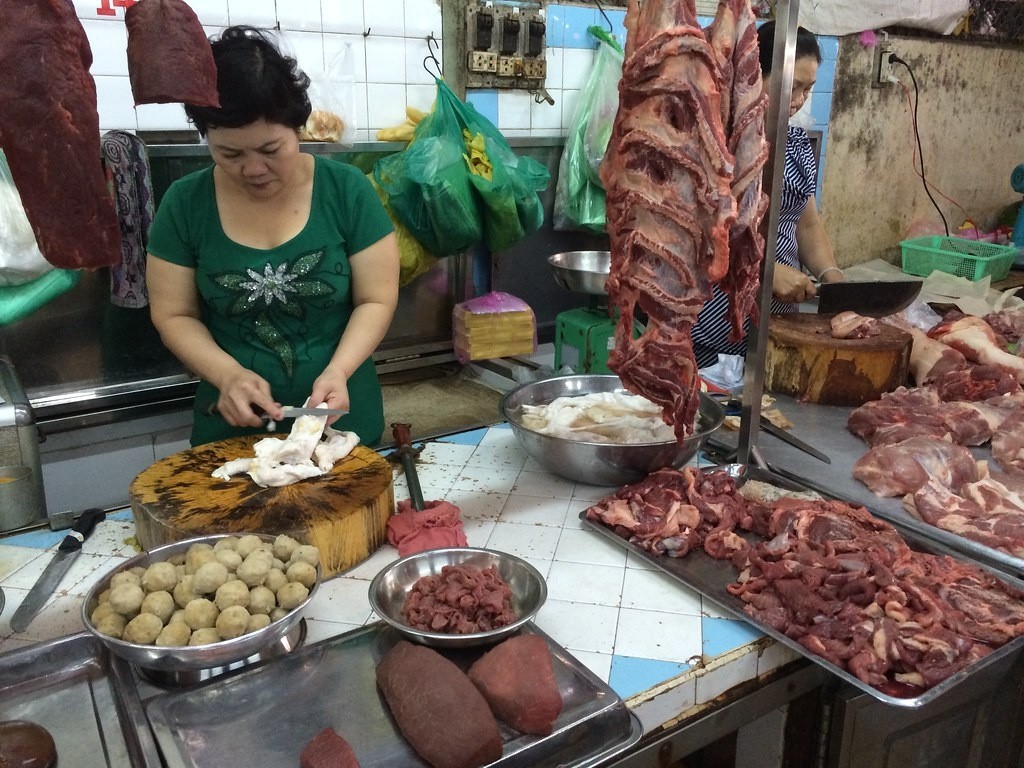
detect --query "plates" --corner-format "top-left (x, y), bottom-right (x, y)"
top-left (146, 620), bottom-right (644, 768)
top-left (577, 461), bottom-right (1024, 708)
top-left (0, 632), bottom-right (162, 768)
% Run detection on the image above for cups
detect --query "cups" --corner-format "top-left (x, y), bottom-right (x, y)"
top-left (0, 465), bottom-right (38, 530)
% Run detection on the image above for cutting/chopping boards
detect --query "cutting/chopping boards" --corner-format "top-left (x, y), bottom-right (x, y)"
top-left (763, 310), bottom-right (915, 408)
top-left (130, 434), bottom-right (395, 582)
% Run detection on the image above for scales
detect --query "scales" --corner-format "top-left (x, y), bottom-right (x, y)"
top-left (545, 251), bottom-right (647, 377)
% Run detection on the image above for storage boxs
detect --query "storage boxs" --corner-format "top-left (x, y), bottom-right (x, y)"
top-left (893, 233), bottom-right (1020, 285)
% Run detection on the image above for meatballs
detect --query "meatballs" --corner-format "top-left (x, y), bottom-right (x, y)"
top-left (90, 535), bottom-right (320, 649)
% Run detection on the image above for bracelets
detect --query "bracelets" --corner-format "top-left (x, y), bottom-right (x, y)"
top-left (816, 267), bottom-right (842, 284)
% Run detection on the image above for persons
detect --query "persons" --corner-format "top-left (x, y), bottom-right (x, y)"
top-left (684, 21), bottom-right (841, 374)
top-left (143, 25), bottom-right (403, 451)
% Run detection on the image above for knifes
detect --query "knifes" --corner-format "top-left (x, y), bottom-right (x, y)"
top-left (198, 400), bottom-right (350, 419)
top-left (810, 281), bottom-right (924, 317)
top-left (729, 399), bottom-right (830, 465)
top-left (11, 508), bottom-right (106, 632)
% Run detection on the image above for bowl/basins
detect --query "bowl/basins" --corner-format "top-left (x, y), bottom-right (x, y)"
top-left (81, 533), bottom-right (323, 672)
top-left (368, 547), bottom-right (549, 647)
top-left (547, 251), bottom-right (612, 297)
top-left (498, 374), bottom-right (728, 490)
